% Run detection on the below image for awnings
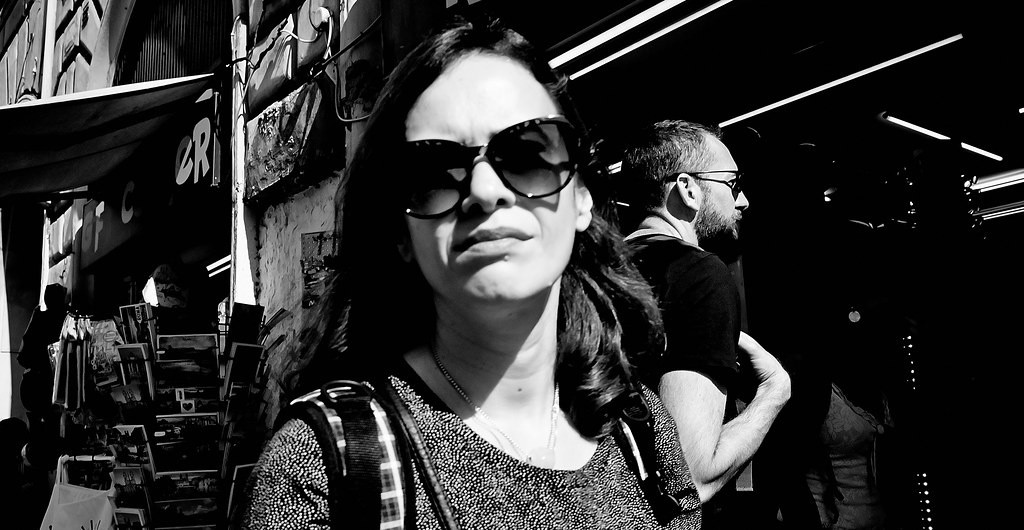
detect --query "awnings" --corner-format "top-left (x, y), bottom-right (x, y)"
top-left (0, 67), bottom-right (218, 209)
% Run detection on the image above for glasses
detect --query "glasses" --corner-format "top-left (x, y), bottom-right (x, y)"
top-left (665, 168), bottom-right (745, 192)
top-left (393, 116), bottom-right (588, 220)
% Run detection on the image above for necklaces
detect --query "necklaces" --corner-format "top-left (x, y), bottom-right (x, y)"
top-left (426, 343), bottom-right (563, 476)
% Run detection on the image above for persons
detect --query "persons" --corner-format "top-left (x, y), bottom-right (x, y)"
top-left (241, 16), bottom-right (706, 530)
top-left (21, 281), bottom-right (68, 443)
top-left (585, 116), bottom-right (898, 530)
